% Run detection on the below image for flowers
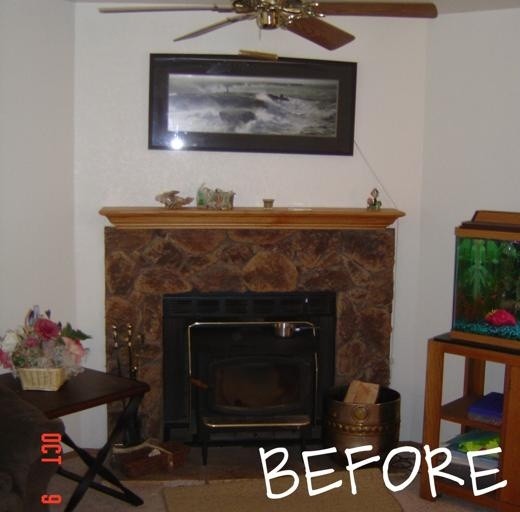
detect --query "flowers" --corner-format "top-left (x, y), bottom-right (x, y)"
top-left (0, 305), bottom-right (93, 371)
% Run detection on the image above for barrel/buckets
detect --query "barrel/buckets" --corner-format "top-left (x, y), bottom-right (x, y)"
top-left (322, 385), bottom-right (401, 469)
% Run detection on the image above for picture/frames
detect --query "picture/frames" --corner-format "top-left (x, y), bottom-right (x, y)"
top-left (148, 52), bottom-right (358, 156)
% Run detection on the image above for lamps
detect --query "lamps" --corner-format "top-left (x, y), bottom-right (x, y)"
top-left (232, 0), bottom-right (326, 30)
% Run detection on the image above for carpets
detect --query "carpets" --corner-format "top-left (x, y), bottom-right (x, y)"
top-left (162, 467), bottom-right (404, 511)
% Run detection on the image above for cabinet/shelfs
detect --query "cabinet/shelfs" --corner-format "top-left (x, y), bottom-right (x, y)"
top-left (419, 332), bottom-right (520, 512)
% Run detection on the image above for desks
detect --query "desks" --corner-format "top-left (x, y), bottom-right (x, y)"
top-left (0, 366), bottom-right (152, 512)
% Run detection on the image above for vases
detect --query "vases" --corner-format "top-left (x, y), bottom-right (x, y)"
top-left (17, 366), bottom-right (68, 392)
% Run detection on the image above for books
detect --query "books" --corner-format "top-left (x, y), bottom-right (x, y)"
top-left (435, 428), bottom-right (500, 496)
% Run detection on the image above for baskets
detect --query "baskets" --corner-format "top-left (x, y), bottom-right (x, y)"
top-left (18, 367), bottom-right (64, 391)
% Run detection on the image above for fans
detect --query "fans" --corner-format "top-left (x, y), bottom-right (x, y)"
top-left (99, 0), bottom-right (438, 52)
